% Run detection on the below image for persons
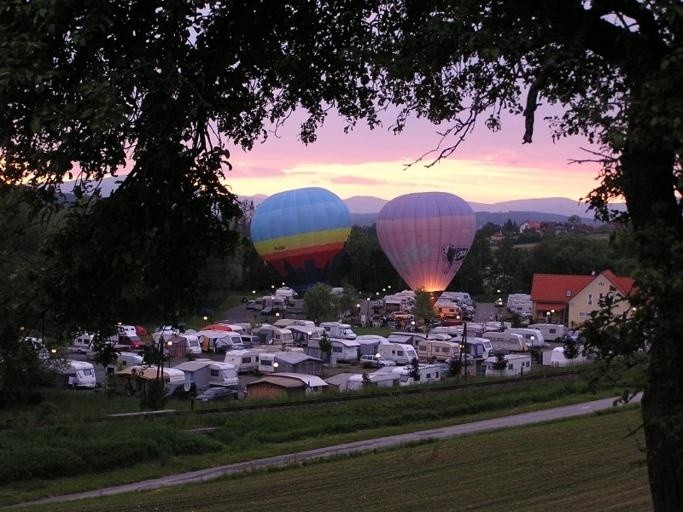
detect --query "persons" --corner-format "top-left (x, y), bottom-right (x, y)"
top-left (361, 312), bottom-right (405, 332)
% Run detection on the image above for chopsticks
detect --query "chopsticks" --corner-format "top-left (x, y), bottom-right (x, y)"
top-left (251, 187), bottom-right (353, 326)
top-left (376, 192), bottom-right (477, 320)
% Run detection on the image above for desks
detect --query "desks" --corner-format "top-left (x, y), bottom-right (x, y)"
top-left (356, 285), bottom-right (391, 324)
top-left (203, 317), bottom-right (207, 339)
top-left (546, 310), bottom-right (554, 324)
top-left (167, 342), bottom-right (172, 367)
top-left (496, 290), bottom-right (503, 325)
top-left (252, 282), bottom-right (285, 316)
top-left (274, 362), bottom-right (279, 375)
top-left (530, 335), bottom-right (535, 361)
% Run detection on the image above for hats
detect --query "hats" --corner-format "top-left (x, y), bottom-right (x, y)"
top-left (194, 386), bottom-right (238, 401)
top-left (360, 354), bottom-right (396, 367)
